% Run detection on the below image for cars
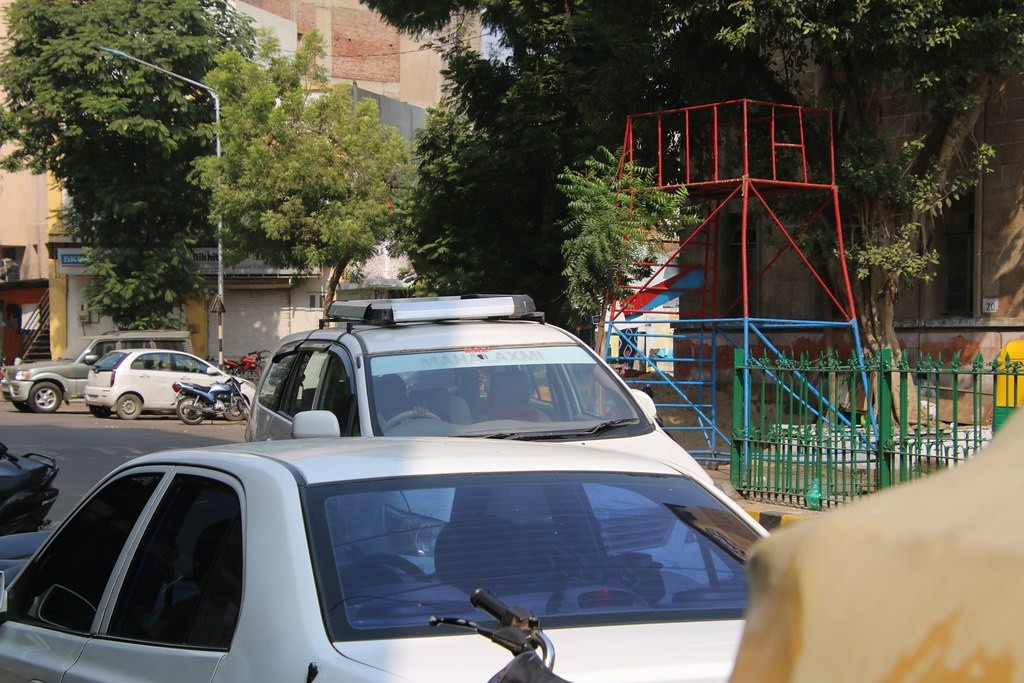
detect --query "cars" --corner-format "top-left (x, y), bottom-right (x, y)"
top-left (0, 438), bottom-right (773, 682)
top-left (84, 348), bottom-right (256, 420)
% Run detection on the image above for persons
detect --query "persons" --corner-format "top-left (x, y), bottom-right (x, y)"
top-left (376, 374), bottom-right (427, 426)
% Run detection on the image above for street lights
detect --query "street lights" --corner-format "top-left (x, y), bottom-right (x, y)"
top-left (97, 46), bottom-right (224, 307)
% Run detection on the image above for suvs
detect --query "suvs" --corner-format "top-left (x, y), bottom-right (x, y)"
top-left (1, 329), bottom-right (194, 414)
top-left (245, 294), bottom-right (728, 495)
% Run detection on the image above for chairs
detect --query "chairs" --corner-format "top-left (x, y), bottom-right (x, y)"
top-left (144, 357), bottom-right (155, 369)
top-left (432, 516), bottom-right (584, 592)
top-left (481, 370), bottom-right (552, 423)
top-left (157, 518), bottom-right (241, 644)
top-left (409, 370), bottom-right (473, 425)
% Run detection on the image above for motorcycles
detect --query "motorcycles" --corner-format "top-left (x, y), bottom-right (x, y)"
top-left (0, 441), bottom-right (60, 536)
top-left (211, 348), bottom-right (271, 382)
top-left (170, 375), bottom-right (250, 425)
